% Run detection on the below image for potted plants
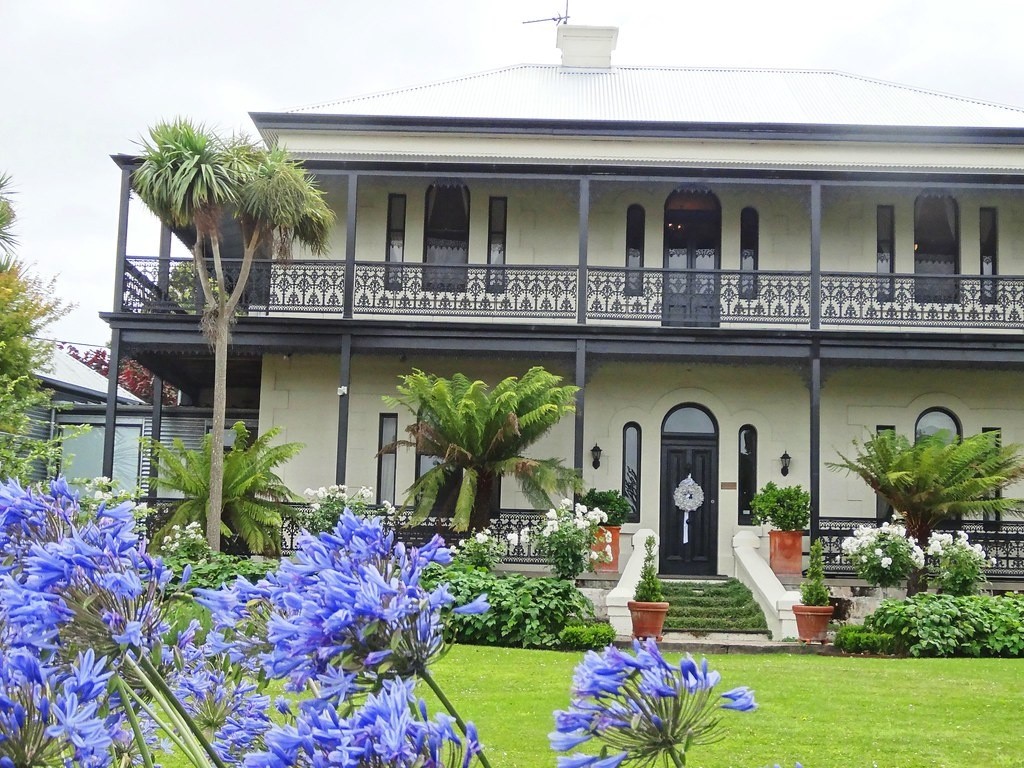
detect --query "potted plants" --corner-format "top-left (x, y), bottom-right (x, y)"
top-left (749, 481), bottom-right (811, 575)
top-left (577, 489), bottom-right (632, 573)
top-left (625, 534), bottom-right (670, 641)
top-left (792, 536), bottom-right (834, 644)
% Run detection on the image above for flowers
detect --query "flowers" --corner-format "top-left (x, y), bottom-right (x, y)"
top-left (673, 484), bottom-right (705, 512)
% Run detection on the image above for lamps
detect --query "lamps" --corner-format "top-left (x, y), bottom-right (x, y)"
top-left (780, 451), bottom-right (791, 476)
top-left (590, 443), bottom-right (603, 469)
top-left (282, 354), bottom-right (294, 362)
top-left (397, 352), bottom-right (408, 363)
